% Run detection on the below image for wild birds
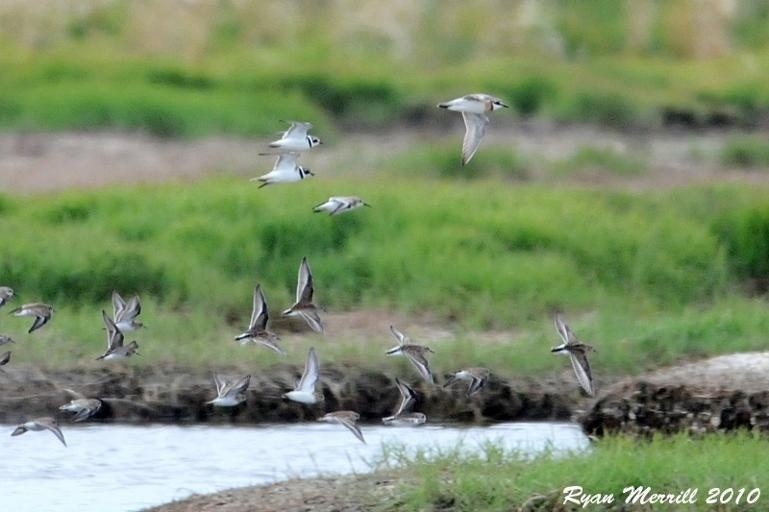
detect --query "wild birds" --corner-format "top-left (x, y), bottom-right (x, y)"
top-left (436, 92), bottom-right (510, 168)
top-left (204, 369), bottom-right (251, 407)
top-left (232, 255), bottom-right (326, 354)
top-left (10, 386), bottom-right (104, 448)
top-left (249, 117), bottom-right (324, 190)
top-left (316, 409), bottom-right (367, 445)
top-left (0, 285), bottom-right (58, 366)
top-left (381, 323), bottom-right (493, 429)
top-left (550, 310), bottom-right (597, 399)
top-left (312, 196), bottom-right (372, 217)
top-left (279, 348), bottom-right (325, 406)
top-left (96, 289), bottom-right (147, 363)
